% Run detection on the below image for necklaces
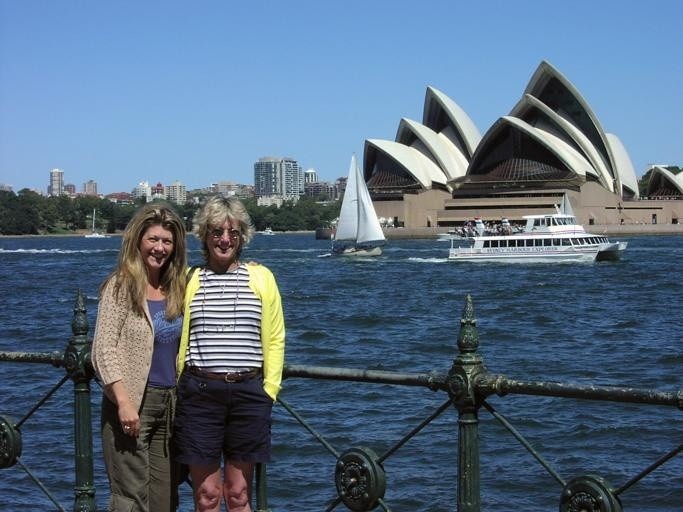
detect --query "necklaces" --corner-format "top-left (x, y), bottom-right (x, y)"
top-left (209, 263), bottom-right (238, 295)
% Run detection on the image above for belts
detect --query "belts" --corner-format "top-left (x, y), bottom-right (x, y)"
top-left (190, 367), bottom-right (258, 383)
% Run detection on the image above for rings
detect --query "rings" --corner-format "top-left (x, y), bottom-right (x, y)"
top-left (123, 424), bottom-right (131, 433)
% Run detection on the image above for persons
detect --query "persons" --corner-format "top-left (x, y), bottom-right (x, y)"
top-left (454, 220), bottom-right (523, 236)
top-left (175, 195), bottom-right (285, 512)
top-left (90, 204), bottom-right (187, 512)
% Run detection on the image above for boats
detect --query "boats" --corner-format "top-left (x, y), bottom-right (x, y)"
top-left (444, 214), bottom-right (628, 265)
top-left (436, 223), bottom-right (521, 241)
top-left (263, 226), bottom-right (273, 235)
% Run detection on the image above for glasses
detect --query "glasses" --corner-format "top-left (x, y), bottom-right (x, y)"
top-left (208, 226), bottom-right (241, 240)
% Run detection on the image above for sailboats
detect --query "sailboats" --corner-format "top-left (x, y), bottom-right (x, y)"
top-left (324, 152), bottom-right (389, 257)
top-left (84, 209), bottom-right (112, 238)
